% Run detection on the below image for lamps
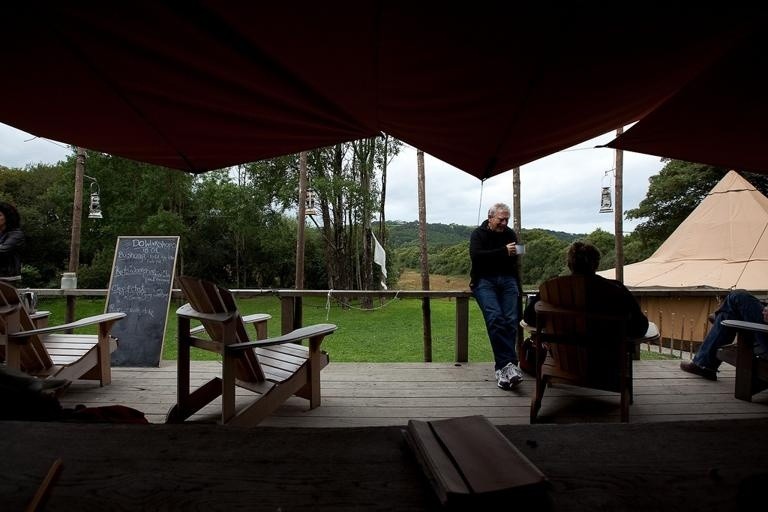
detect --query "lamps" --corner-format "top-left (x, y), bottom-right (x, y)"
top-left (600, 167), bottom-right (616, 214)
top-left (88, 182), bottom-right (103, 219)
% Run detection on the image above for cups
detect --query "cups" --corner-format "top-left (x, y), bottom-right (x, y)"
top-left (512, 244), bottom-right (526, 255)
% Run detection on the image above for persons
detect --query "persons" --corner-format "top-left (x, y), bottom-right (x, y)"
top-left (679, 288), bottom-right (767, 380)
top-left (518, 241), bottom-right (649, 363)
top-left (468, 203), bottom-right (523, 391)
top-left (0, 202), bottom-right (24, 288)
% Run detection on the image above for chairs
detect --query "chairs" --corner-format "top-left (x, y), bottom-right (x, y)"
top-left (165, 275), bottom-right (339, 430)
top-left (716, 320), bottom-right (768, 403)
top-left (0, 280), bottom-right (128, 387)
top-left (530, 274), bottom-right (633, 423)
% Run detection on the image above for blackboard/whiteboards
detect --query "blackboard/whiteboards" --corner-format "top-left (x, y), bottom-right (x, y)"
top-left (103, 236), bottom-right (180, 369)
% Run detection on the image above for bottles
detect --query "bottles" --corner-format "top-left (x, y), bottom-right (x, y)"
top-left (60, 272), bottom-right (78, 289)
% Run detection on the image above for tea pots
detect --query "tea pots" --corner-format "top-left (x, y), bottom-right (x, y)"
top-left (19, 289), bottom-right (35, 315)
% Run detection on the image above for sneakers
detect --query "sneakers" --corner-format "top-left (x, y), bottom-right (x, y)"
top-left (494, 365), bottom-right (509, 390)
top-left (678, 356), bottom-right (717, 382)
top-left (504, 361), bottom-right (524, 389)
top-left (708, 309), bottom-right (715, 323)
